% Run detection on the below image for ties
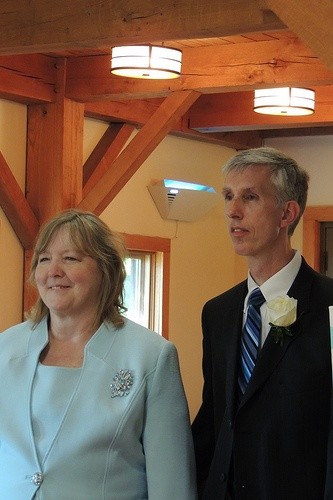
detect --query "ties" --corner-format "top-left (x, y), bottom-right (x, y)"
top-left (233, 285), bottom-right (267, 407)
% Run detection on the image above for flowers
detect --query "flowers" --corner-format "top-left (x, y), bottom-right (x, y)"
top-left (266, 295), bottom-right (298, 347)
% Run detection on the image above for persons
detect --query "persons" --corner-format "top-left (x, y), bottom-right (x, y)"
top-left (0, 206), bottom-right (198, 500)
top-left (190, 145), bottom-right (333, 500)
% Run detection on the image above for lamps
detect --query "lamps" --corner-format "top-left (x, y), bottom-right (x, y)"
top-left (110, 44), bottom-right (183, 79)
top-left (253, 86), bottom-right (315, 117)
